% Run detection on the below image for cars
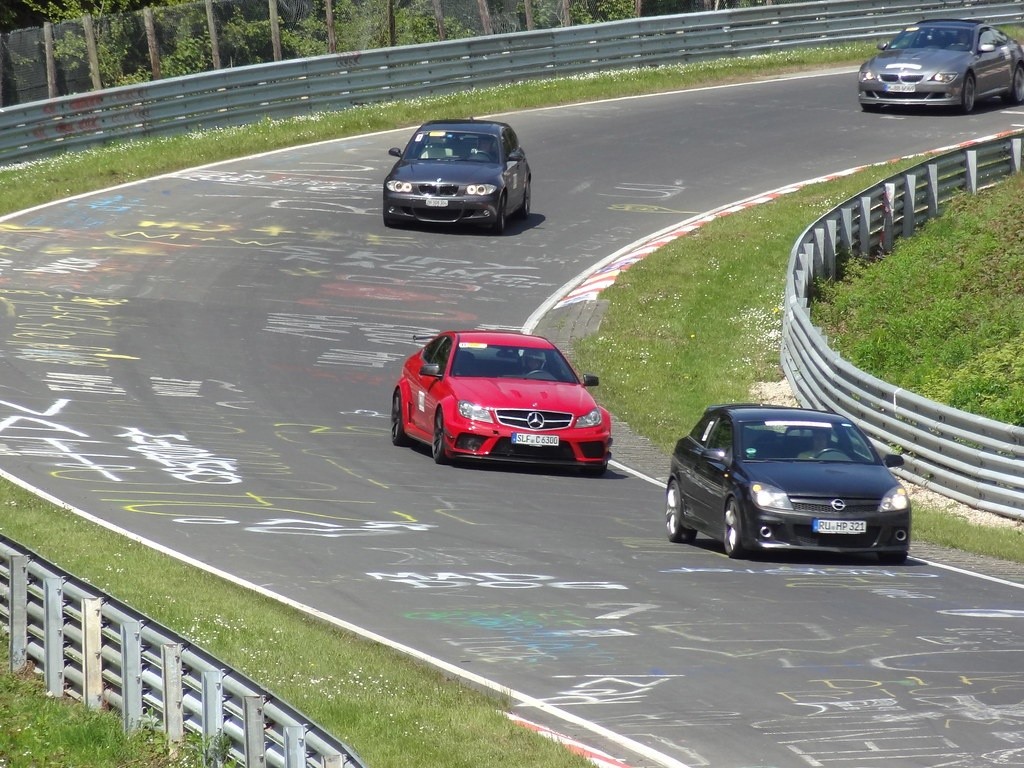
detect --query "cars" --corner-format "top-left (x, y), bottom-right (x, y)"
top-left (855, 17), bottom-right (1024, 112)
top-left (665, 402), bottom-right (913, 564)
top-left (381, 117), bottom-right (532, 234)
top-left (390, 329), bottom-right (613, 474)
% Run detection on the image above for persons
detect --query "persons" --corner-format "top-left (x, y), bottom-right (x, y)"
top-left (459, 135), bottom-right (499, 162)
top-left (949, 30), bottom-right (973, 48)
top-left (516, 348), bottom-right (549, 378)
top-left (794, 428), bottom-right (833, 461)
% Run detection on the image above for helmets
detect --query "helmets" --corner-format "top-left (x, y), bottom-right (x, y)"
top-left (521, 351), bottom-right (547, 371)
